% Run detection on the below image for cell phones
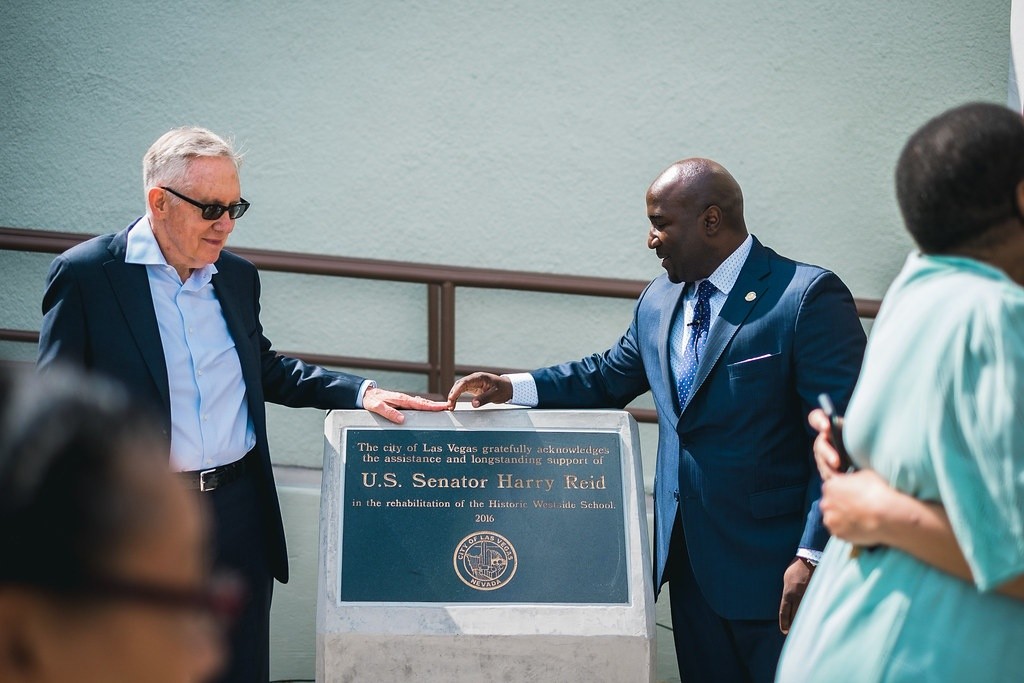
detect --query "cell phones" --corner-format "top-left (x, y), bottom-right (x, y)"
top-left (818, 392), bottom-right (855, 474)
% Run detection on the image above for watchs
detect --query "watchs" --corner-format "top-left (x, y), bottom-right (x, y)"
top-left (809, 559), bottom-right (818, 568)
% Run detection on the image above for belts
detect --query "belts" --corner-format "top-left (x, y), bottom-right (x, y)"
top-left (172, 449), bottom-right (261, 491)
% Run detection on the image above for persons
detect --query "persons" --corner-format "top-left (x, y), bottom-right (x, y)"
top-left (0, 365), bottom-right (244, 683)
top-left (772, 100), bottom-right (1024, 683)
top-left (445, 160), bottom-right (871, 683)
top-left (38, 128), bottom-right (449, 683)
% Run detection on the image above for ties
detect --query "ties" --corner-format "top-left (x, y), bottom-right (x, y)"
top-left (673, 281), bottom-right (717, 413)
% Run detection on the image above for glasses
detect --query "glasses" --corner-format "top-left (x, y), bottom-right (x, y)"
top-left (161, 186), bottom-right (250, 221)
top-left (92, 568), bottom-right (248, 620)
